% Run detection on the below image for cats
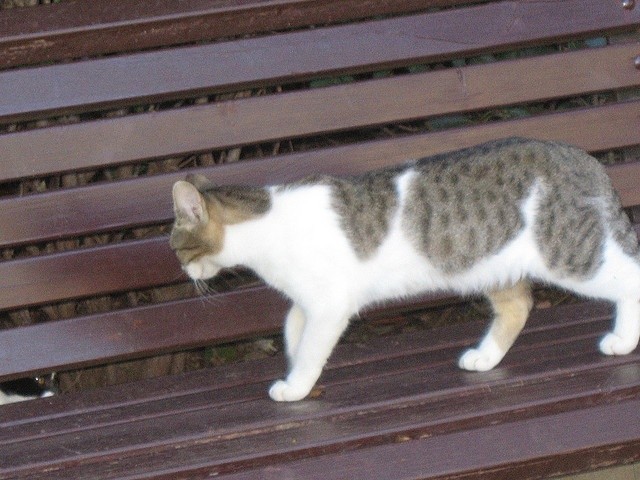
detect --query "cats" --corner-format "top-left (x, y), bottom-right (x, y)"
top-left (165, 137), bottom-right (640, 403)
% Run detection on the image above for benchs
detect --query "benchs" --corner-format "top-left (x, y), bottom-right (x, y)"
top-left (2, 2), bottom-right (640, 479)
